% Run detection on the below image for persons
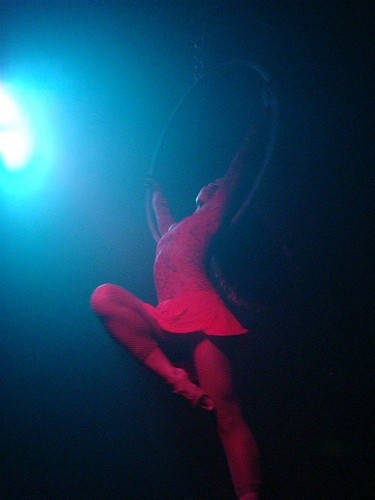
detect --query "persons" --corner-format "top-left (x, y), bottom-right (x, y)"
top-left (88, 77), bottom-right (275, 500)
